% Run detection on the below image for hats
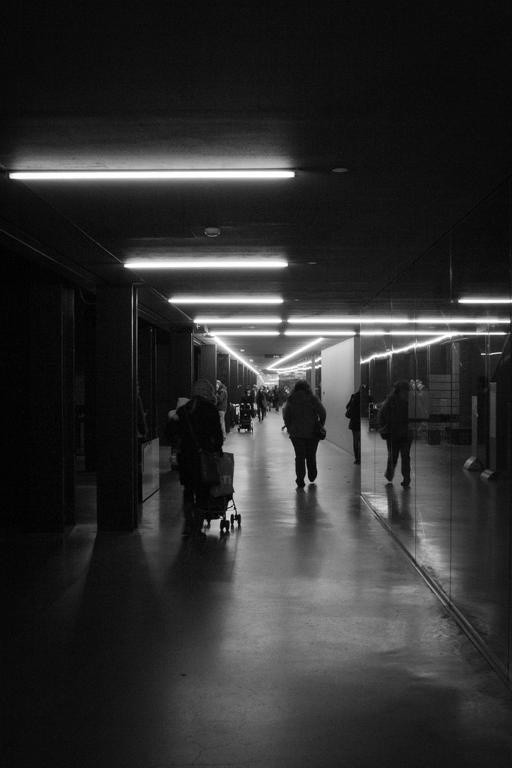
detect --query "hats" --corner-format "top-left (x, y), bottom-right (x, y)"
top-left (192, 378), bottom-right (217, 406)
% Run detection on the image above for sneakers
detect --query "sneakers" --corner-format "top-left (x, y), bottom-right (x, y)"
top-left (181, 524), bottom-right (207, 539)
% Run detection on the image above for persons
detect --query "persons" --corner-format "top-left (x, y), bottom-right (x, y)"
top-left (283, 380), bottom-right (326, 488)
top-left (229, 384), bottom-right (289, 432)
top-left (168, 379), bottom-right (224, 539)
top-left (345, 390), bottom-right (360, 464)
top-left (216, 380), bottom-right (228, 441)
top-left (378, 382), bottom-right (414, 486)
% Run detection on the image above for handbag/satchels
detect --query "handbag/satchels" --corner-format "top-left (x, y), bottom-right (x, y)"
top-left (380, 423), bottom-right (393, 440)
top-left (313, 424), bottom-right (326, 439)
top-left (198, 451), bottom-right (220, 490)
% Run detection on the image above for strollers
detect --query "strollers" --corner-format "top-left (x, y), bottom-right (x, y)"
top-left (238, 409), bottom-right (253, 432)
top-left (190, 452), bottom-right (241, 538)
